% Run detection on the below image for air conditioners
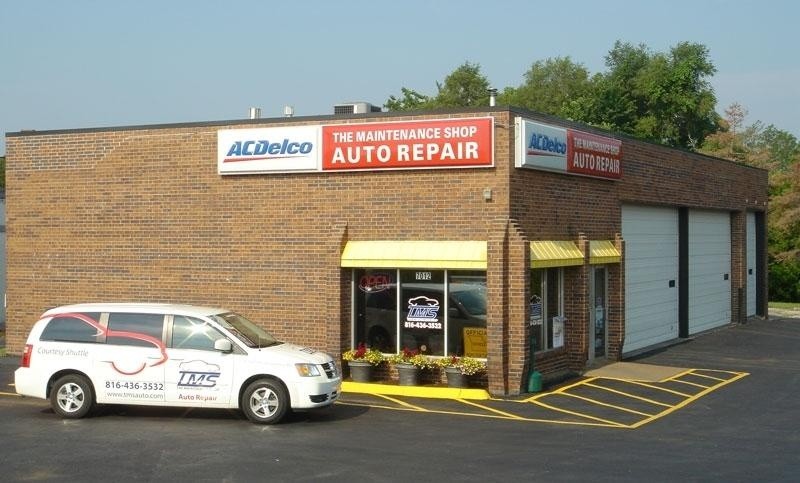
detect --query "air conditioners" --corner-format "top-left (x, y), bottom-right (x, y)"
top-left (333, 102), bottom-right (382, 116)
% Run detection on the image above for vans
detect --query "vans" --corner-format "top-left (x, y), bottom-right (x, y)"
top-left (14, 303), bottom-right (341, 425)
top-left (367, 282), bottom-right (485, 356)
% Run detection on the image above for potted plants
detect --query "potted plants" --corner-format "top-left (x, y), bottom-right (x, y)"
top-left (385, 344), bottom-right (434, 386)
top-left (341, 340), bottom-right (384, 383)
top-left (435, 355), bottom-right (466, 388)
top-left (460, 357), bottom-right (488, 388)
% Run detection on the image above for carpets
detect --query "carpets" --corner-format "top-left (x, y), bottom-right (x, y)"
top-left (583, 360), bottom-right (690, 383)
